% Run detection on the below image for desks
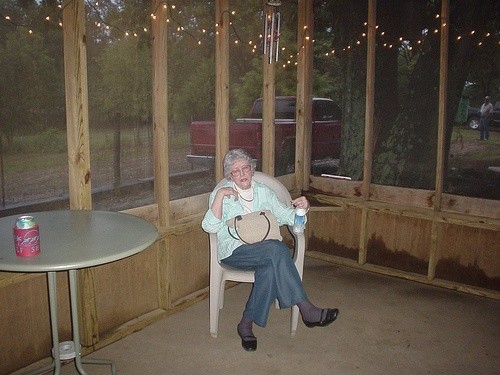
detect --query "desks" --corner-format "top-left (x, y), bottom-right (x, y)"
top-left (0, 211), bottom-right (159, 375)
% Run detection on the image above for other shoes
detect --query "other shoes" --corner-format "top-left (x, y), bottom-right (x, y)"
top-left (477, 137), bottom-right (483, 140)
top-left (301, 308), bottom-right (339, 329)
top-left (484, 138), bottom-right (488, 140)
top-left (237, 323), bottom-right (258, 351)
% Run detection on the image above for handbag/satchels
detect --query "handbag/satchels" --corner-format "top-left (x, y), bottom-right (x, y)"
top-left (225, 209), bottom-right (283, 246)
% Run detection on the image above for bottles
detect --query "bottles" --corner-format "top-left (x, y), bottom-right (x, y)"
top-left (292, 206), bottom-right (307, 234)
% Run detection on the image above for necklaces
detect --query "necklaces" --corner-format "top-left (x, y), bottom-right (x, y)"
top-left (235, 185), bottom-right (254, 202)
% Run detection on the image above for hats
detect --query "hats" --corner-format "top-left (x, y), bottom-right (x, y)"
top-left (485, 96), bottom-right (491, 100)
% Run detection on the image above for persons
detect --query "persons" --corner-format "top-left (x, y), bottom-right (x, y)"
top-left (478, 96), bottom-right (493, 141)
top-left (201, 148), bottom-right (339, 351)
top-left (493, 97), bottom-right (500, 121)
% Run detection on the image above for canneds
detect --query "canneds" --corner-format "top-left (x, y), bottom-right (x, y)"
top-left (15, 216), bottom-right (37, 229)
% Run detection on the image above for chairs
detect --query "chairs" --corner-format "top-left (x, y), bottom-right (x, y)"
top-left (208, 171), bottom-right (305, 337)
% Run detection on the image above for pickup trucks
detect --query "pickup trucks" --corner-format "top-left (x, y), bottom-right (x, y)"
top-left (187, 95), bottom-right (343, 175)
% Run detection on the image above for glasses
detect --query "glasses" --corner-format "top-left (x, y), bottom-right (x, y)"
top-left (230, 165), bottom-right (251, 177)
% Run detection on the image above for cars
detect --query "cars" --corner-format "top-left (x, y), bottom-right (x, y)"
top-left (467, 102), bottom-right (500, 130)
